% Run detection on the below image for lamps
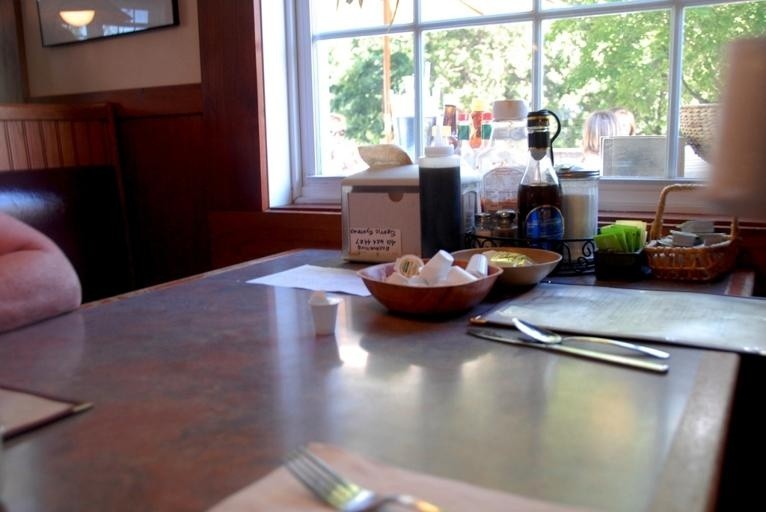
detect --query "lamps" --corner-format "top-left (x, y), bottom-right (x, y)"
top-left (37, 0), bottom-right (131, 28)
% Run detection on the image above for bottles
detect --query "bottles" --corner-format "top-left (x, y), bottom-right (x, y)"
top-left (434, 92), bottom-right (459, 149)
top-left (453, 107), bottom-right (473, 160)
top-left (471, 213), bottom-right (496, 248)
top-left (417, 126), bottom-right (460, 258)
top-left (517, 108), bottom-right (564, 243)
top-left (551, 163), bottom-right (600, 249)
top-left (492, 208), bottom-right (518, 248)
top-left (474, 98), bottom-right (535, 216)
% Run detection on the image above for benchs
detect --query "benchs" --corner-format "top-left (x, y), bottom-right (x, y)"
top-left (0, 161), bottom-right (181, 311)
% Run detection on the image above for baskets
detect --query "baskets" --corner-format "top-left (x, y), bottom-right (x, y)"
top-left (643, 183), bottom-right (743, 281)
top-left (680, 104), bottom-right (726, 169)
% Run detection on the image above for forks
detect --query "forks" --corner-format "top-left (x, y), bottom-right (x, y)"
top-left (271, 440), bottom-right (439, 512)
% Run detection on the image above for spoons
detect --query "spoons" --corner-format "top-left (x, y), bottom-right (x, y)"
top-left (511, 316), bottom-right (670, 360)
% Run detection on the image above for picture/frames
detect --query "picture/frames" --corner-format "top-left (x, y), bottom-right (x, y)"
top-left (32, 0), bottom-right (178, 53)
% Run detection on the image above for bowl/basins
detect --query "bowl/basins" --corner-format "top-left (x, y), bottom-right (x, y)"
top-left (353, 258), bottom-right (501, 318)
top-left (460, 247), bottom-right (564, 290)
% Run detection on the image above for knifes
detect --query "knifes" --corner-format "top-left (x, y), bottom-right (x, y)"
top-left (468, 328), bottom-right (668, 374)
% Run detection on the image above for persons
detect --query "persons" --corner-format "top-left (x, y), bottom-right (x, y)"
top-left (0, 212), bottom-right (82, 333)
top-left (615, 109), bottom-right (635, 136)
top-left (579, 110), bottom-right (618, 170)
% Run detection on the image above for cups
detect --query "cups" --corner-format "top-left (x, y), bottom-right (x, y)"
top-left (309, 296), bottom-right (343, 337)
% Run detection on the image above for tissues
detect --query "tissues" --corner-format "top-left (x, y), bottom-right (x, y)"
top-left (339, 142), bottom-right (421, 264)
